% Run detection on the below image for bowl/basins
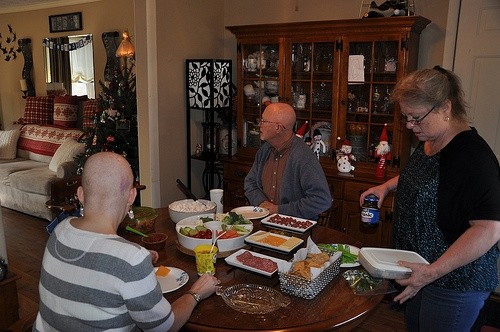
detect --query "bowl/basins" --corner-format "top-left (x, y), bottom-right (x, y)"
top-left (216, 284), bottom-right (291, 314)
top-left (176, 213), bottom-right (253, 252)
top-left (142, 233), bottom-right (168, 251)
top-left (359, 247), bottom-right (430, 279)
top-left (121, 206), bottom-right (159, 234)
top-left (169, 199), bottom-right (217, 223)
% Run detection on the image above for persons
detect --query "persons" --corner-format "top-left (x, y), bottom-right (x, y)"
top-left (360, 64), bottom-right (500, 332)
top-left (31, 151), bottom-right (222, 332)
top-left (244, 102), bottom-right (333, 221)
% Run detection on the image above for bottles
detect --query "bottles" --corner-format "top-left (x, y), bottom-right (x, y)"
top-left (289, 45), bottom-right (394, 117)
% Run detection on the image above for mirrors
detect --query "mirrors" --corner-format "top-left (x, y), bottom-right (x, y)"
top-left (43, 34), bottom-right (96, 99)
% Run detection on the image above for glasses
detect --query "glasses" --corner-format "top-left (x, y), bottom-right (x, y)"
top-left (399, 105), bottom-right (436, 125)
top-left (261, 118), bottom-right (286, 129)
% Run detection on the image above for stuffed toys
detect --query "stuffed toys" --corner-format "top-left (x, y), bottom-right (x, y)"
top-left (309, 129), bottom-right (326, 160)
top-left (374, 126), bottom-right (394, 177)
top-left (336, 140), bottom-right (356, 173)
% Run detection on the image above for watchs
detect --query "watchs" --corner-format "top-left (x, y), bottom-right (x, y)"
top-left (184, 291), bottom-right (201, 302)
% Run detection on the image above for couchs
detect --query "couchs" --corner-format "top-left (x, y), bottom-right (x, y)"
top-left (0, 122), bottom-right (87, 222)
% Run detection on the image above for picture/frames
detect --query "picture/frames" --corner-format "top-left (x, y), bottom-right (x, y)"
top-left (49, 12), bottom-right (82, 33)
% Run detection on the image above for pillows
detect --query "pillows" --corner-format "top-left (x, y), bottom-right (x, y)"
top-left (0, 130), bottom-right (21, 160)
top-left (53, 96), bottom-right (80, 127)
top-left (22, 97), bottom-right (53, 124)
top-left (49, 139), bottom-right (86, 172)
top-left (80, 99), bottom-right (105, 129)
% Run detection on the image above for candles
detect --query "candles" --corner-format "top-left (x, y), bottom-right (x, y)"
top-left (20, 79), bottom-right (27, 90)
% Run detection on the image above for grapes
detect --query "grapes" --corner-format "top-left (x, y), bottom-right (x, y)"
top-left (193, 229), bottom-right (212, 239)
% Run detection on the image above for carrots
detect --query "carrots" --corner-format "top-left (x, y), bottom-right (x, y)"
top-left (218, 230), bottom-right (239, 239)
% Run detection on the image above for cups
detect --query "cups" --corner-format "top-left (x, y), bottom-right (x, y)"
top-left (210, 189), bottom-right (224, 214)
top-left (241, 47), bottom-right (279, 111)
top-left (194, 245), bottom-right (218, 277)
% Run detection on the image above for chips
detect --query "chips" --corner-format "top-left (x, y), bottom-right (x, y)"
top-left (287, 252), bottom-right (329, 288)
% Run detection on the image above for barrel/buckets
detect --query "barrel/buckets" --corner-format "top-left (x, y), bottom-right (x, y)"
top-left (343, 134), bottom-right (368, 160)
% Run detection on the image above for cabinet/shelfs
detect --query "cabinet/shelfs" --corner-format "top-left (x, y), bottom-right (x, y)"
top-left (224, 17), bottom-right (432, 244)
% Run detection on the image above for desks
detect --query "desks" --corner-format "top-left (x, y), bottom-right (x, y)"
top-left (119, 205), bottom-right (387, 332)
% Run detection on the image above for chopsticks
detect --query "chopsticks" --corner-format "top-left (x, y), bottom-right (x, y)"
top-left (176, 178), bottom-right (207, 207)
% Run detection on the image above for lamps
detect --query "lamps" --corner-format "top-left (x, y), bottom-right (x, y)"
top-left (186, 58), bottom-right (232, 194)
top-left (116, 31), bottom-right (134, 81)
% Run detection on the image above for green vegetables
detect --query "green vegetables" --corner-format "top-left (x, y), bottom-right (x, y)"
top-left (221, 211), bottom-right (251, 231)
top-left (199, 217), bottom-right (213, 223)
top-left (317, 243), bottom-right (359, 264)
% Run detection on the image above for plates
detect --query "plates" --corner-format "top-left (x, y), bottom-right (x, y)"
top-left (224, 249), bottom-right (288, 276)
top-left (330, 244), bottom-right (361, 267)
top-left (261, 213), bottom-right (317, 232)
top-left (312, 121), bottom-right (331, 156)
top-left (244, 230), bottom-right (304, 252)
top-left (231, 206), bottom-right (270, 219)
top-left (153, 267), bottom-right (189, 293)
